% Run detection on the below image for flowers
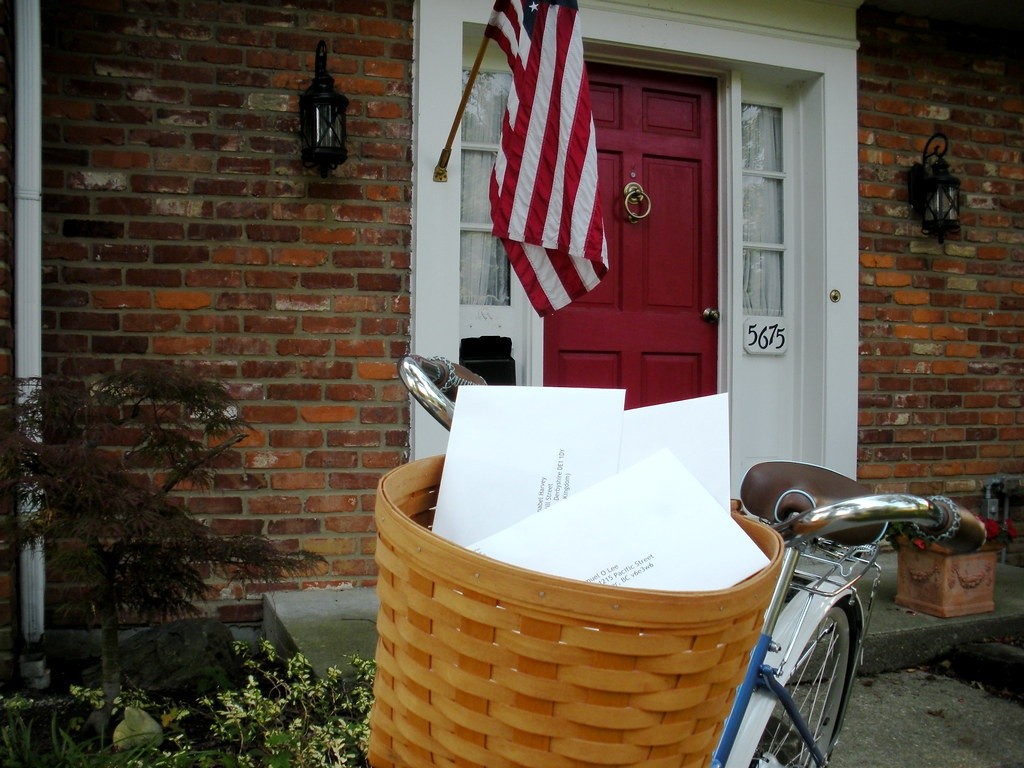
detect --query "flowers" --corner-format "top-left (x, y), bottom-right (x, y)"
top-left (884, 504), bottom-right (1019, 553)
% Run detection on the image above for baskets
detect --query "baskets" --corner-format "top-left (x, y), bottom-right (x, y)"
top-left (368, 453), bottom-right (786, 768)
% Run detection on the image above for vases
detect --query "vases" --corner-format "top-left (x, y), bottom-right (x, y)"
top-left (894, 535), bottom-right (1003, 618)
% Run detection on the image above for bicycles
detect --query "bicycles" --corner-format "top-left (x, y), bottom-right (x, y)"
top-left (396, 349), bottom-right (988, 767)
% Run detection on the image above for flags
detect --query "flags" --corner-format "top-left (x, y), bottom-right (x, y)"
top-left (484, 0), bottom-right (610, 318)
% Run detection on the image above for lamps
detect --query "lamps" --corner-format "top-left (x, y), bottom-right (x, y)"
top-left (909, 131), bottom-right (962, 247)
top-left (297, 39), bottom-right (351, 180)
